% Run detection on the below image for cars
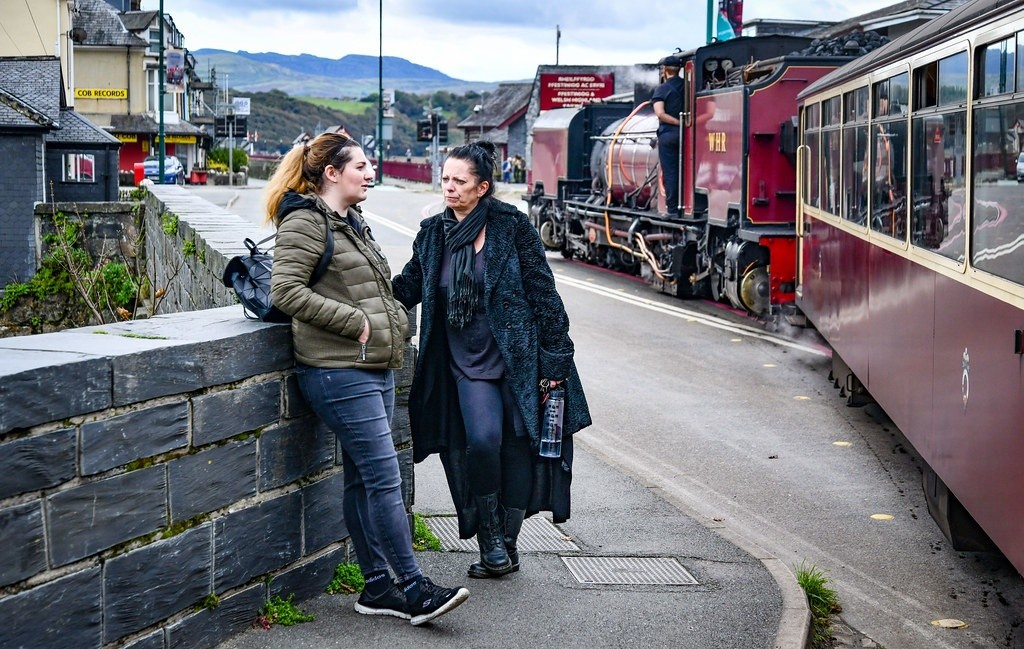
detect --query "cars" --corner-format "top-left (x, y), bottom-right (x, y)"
top-left (142, 154), bottom-right (186, 185)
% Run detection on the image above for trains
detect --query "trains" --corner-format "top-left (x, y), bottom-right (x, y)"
top-left (518, 0), bottom-right (1024, 582)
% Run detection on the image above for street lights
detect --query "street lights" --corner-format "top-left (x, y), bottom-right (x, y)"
top-left (472, 90), bottom-right (485, 140)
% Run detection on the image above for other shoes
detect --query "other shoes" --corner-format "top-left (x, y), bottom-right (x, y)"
top-left (664, 209), bottom-right (679, 216)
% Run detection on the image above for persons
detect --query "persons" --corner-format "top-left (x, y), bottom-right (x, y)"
top-left (404, 149), bottom-right (412, 163)
top-left (391, 139), bottom-right (594, 581)
top-left (502, 154), bottom-right (527, 184)
top-left (259, 129), bottom-right (471, 626)
top-left (651, 55), bottom-right (689, 218)
top-left (696, 96), bottom-right (717, 184)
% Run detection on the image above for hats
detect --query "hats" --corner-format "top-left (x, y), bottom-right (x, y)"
top-left (663, 56), bottom-right (681, 67)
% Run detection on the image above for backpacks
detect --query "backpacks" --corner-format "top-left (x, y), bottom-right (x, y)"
top-left (221, 203), bottom-right (335, 324)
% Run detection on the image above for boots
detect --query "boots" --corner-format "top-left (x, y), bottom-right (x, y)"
top-left (467, 505), bottom-right (526, 578)
top-left (475, 488), bottom-right (513, 573)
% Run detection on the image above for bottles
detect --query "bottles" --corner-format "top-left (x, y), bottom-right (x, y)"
top-left (538, 388), bottom-right (564, 457)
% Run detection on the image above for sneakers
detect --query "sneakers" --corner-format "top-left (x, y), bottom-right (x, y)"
top-left (353, 568), bottom-right (412, 620)
top-left (390, 574), bottom-right (471, 626)
